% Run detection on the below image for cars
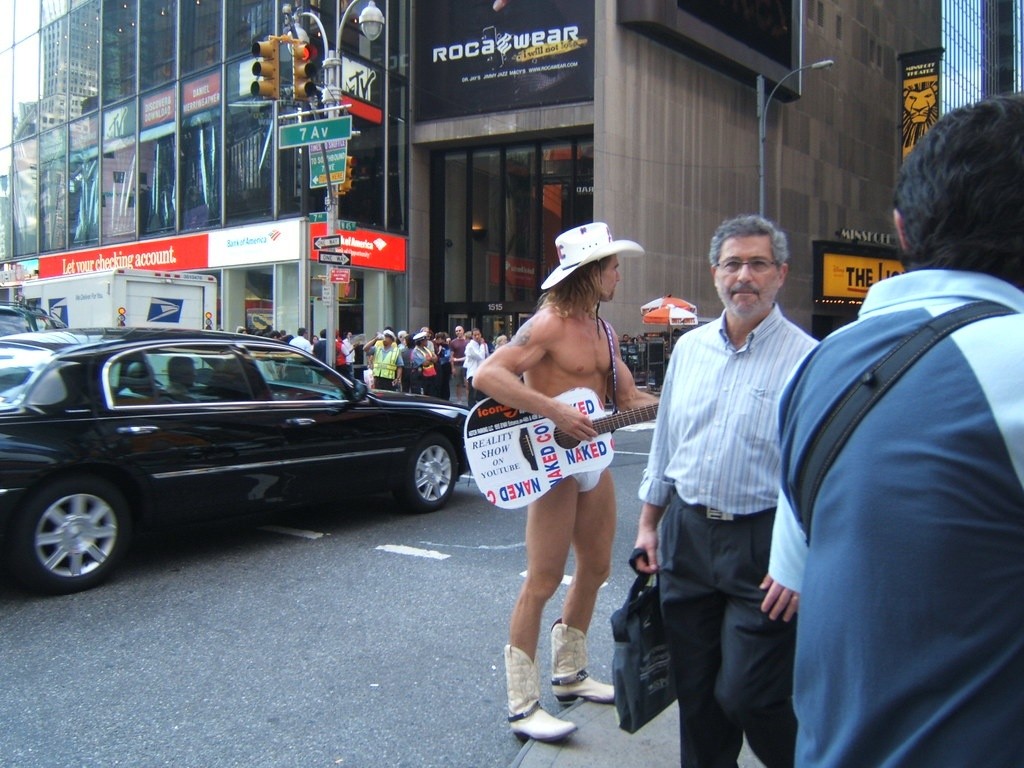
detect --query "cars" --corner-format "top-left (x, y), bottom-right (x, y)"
top-left (0, 300), bottom-right (70, 336)
top-left (0, 326), bottom-right (472, 596)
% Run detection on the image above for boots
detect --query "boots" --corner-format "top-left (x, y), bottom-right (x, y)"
top-left (551, 618), bottom-right (615, 707)
top-left (503, 645), bottom-right (577, 742)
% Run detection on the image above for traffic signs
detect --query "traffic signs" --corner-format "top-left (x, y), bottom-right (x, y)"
top-left (319, 251), bottom-right (352, 266)
top-left (313, 234), bottom-right (342, 250)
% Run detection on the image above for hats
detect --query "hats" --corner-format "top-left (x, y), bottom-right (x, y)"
top-left (541, 221), bottom-right (646, 289)
top-left (412, 332), bottom-right (428, 342)
top-left (383, 330), bottom-right (397, 343)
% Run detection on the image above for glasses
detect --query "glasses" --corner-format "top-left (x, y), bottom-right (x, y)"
top-left (714, 258), bottom-right (775, 274)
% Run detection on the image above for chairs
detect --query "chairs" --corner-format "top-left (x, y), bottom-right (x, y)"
top-left (124, 362), bottom-right (158, 404)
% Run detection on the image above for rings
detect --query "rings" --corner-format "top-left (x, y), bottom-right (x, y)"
top-left (792, 597), bottom-right (799, 600)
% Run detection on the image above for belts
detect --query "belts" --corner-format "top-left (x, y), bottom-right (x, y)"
top-left (676, 495), bottom-right (776, 521)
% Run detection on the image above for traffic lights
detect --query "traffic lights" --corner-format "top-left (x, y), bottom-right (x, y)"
top-left (251, 41), bottom-right (280, 99)
top-left (291, 40), bottom-right (318, 103)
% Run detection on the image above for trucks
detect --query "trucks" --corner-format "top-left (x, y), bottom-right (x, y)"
top-left (0, 267), bottom-right (219, 331)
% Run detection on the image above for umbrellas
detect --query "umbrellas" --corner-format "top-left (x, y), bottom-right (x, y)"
top-left (640, 294), bottom-right (698, 316)
top-left (643, 304), bottom-right (698, 350)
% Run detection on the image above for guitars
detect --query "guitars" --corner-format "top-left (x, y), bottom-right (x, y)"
top-left (461, 385), bottom-right (661, 511)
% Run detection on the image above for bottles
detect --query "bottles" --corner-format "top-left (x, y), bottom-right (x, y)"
top-left (378, 334), bottom-right (385, 340)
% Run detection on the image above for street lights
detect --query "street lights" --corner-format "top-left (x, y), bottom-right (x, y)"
top-left (755, 59), bottom-right (835, 217)
top-left (287, 1), bottom-right (386, 367)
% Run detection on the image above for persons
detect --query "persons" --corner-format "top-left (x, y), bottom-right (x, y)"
top-left (235, 326), bottom-right (513, 409)
top-left (777, 92), bottom-right (1024, 768)
top-left (471, 221), bottom-right (661, 742)
top-left (633, 215), bottom-right (822, 768)
top-left (618, 327), bottom-right (685, 377)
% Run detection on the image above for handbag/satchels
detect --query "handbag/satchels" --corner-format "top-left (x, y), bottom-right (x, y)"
top-left (364, 369), bottom-right (375, 390)
top-left (610, 549), bottom-right (677, 734)
